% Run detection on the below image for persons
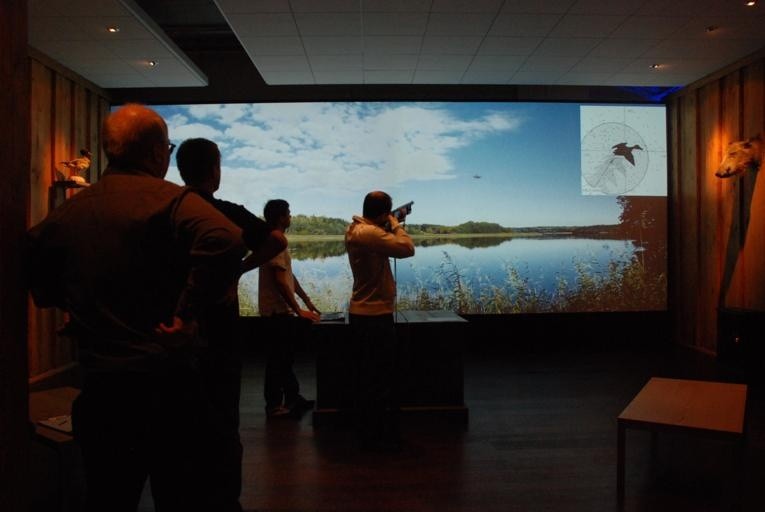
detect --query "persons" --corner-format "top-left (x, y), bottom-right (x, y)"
top-left (173, 139), bottom-right (288, 512)
top-left (258, 200), bottom-right (322, 419)
top-left (25, 101), bottom-right (243, 512)
top-left (342, 191), bottom-right (415, 453)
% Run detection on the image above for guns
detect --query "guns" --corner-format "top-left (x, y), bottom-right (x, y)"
top-left (383, 201), bottom-right (415, 231)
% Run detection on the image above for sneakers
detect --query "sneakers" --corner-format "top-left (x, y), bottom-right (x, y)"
top-left (267, 406), bottom-right (298, 418)
top-left (285, 395), bottom-right (315, 410)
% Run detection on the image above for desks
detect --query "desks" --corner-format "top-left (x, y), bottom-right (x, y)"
top-left (612, 372), bottom-right (756, 509)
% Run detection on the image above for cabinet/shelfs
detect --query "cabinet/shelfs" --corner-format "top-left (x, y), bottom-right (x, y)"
top-left (310, 308), bottom-right (474, 430)
